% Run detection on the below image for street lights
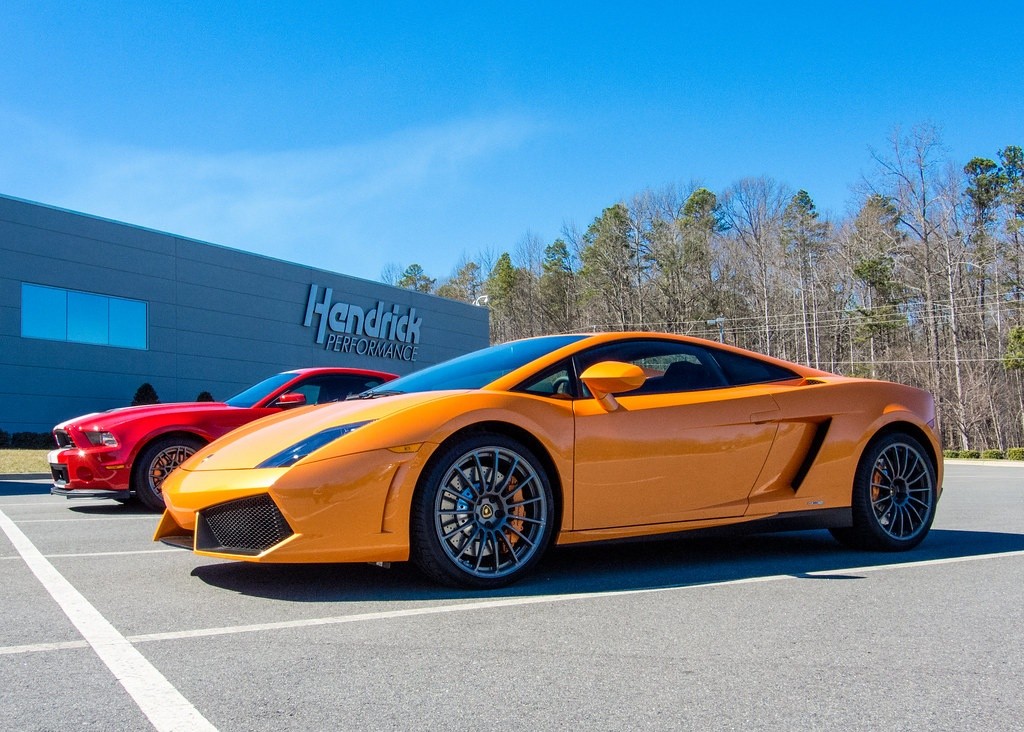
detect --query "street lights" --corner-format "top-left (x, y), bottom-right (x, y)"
top-left (706, 317), bottom-right (725, 343)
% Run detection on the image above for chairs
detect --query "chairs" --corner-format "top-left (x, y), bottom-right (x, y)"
top-left (641, 362), bottom-right (708, 393)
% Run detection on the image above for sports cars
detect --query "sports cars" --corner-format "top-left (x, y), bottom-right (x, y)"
top-left (47, 367), bottom-right (402, 514)
top-left (153, 329), bottom-right (948, 592)
